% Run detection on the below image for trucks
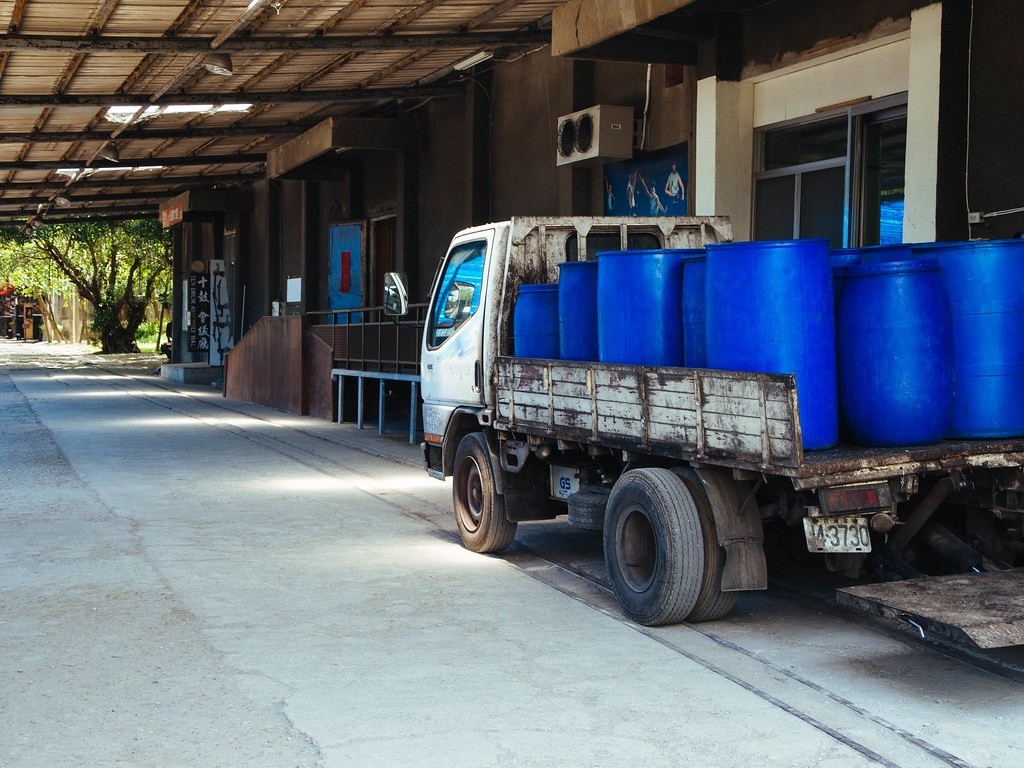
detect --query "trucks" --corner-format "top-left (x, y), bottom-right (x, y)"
top-left (382, 215), bottom-right (1024, 650)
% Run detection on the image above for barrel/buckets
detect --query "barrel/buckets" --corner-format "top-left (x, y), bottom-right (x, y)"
top-left (514, 238), bottom-right (1023, 448)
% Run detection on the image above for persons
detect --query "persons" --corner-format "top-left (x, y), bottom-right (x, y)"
top-left (605, 160), bottom-right (684, 215)
top-left (386, 282), bottom-right (399, 309)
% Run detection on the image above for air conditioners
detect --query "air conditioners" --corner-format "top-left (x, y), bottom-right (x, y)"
top-left (555, 104), bottom-right (635, 169)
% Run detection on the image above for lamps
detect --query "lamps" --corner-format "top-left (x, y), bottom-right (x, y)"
top-left (453, 50), bottom-right (495, 71)
top-left (200, 54), bottom-right (232, 76)
top-left (100, 141), bottom-right (120, 163)
top-left (55, 191), bottom-right (72, 207)
top-left (30, 215), bottom-right (44, 228)
